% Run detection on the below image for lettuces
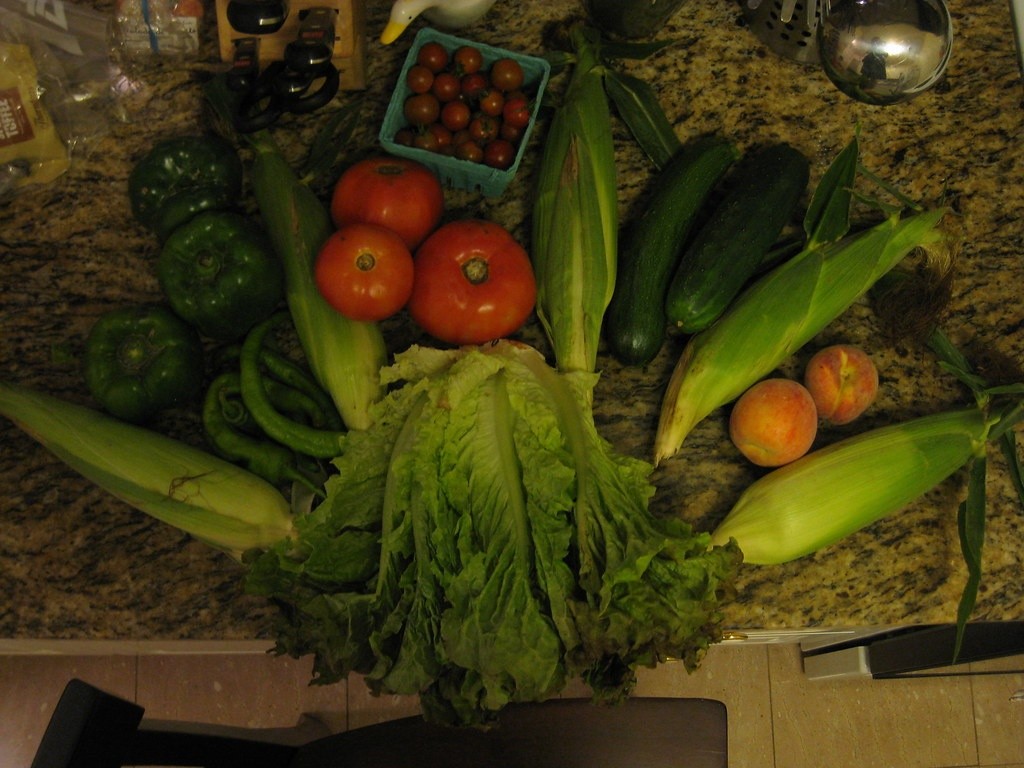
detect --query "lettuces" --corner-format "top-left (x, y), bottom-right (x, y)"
top-left (234, 334), bottom-right (756, 732)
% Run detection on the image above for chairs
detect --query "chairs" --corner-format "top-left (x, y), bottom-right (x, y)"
top-left (29, 679), bottom-right (730, 768)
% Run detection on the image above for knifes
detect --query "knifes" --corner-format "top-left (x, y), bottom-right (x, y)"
top-left (226, 0), bottom-right (338, 102)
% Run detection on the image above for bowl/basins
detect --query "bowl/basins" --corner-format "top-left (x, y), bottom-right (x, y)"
top-left (379, 27), bottom-right (550, 197)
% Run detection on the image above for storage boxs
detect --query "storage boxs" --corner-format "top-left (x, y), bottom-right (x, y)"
top-left (377, 28), bottom-right (551, 197)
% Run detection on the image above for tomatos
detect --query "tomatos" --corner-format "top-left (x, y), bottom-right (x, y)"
top-left (315, 155), bottom-right (535, 347)
top-left (385, 44), bottom-right (532, 169)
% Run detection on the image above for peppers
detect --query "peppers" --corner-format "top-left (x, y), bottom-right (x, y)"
top-left (83, 131), bottom-right (349, 497)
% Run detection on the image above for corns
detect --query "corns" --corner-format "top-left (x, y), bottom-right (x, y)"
top-left (534, 28), bottom-right (621, 388)
top-left (708, 390), bottom-right (1001, 565)
top-left (252, 142), bottom-right (386, 433)
top-left (0, 382), bottom-right (303, 551)
top-left (657, 204), bottom-right (948, 458)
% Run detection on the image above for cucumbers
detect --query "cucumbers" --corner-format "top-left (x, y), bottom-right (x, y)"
top-left (607, 135), bottom-right (809, 363)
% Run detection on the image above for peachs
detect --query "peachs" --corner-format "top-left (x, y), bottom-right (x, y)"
top-left (806, 345), bottom-right (877, 425)
top-left (730, 377), bottom-right (818, 467)
